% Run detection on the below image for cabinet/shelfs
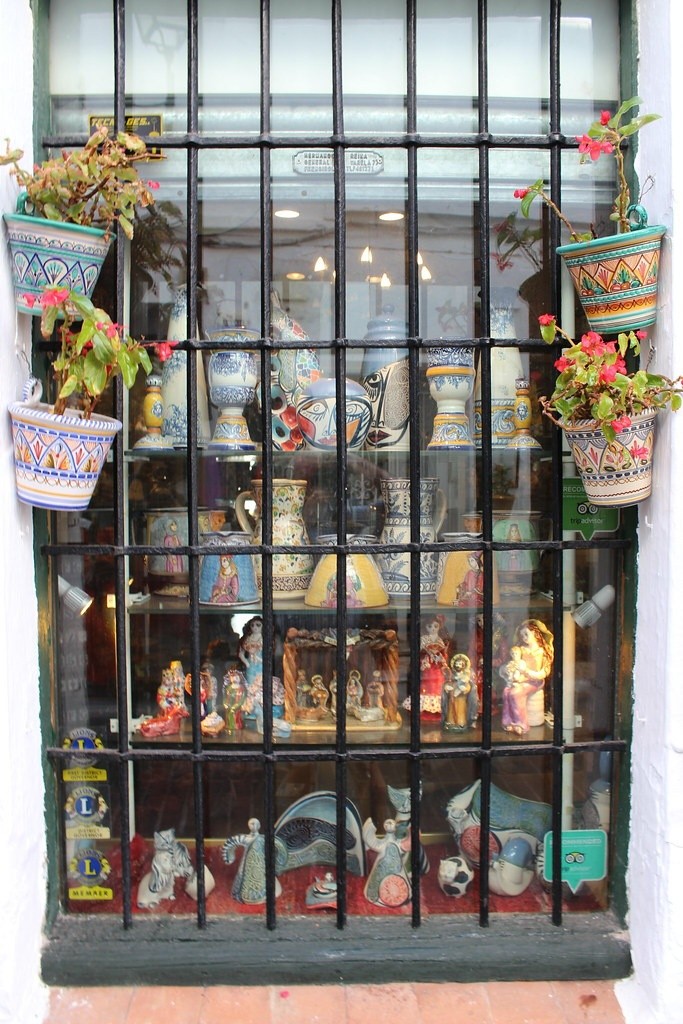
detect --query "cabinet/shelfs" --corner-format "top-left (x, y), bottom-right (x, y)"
top-left (68, 448), bottom-right (619, 920)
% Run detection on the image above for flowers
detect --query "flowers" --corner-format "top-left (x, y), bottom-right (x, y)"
top-left (513, 96), bottom-right (661, 243)
top-left (537, 314), bottom-right (683, 444)
top-left (0, 124), bottom-right (169, 243)
top-left (19, 285), bottom-right (178, 420)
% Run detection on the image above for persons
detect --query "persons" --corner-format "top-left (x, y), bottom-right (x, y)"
top-left (237, 617), bottom-right (262, 716)
top-left (400, 614), bottom-right (555, 736)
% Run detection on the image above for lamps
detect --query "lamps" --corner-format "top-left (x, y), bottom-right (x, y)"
top-left (569, 585), bottom-right (616, 630)
top-left (57, 575), bottom-right (95, 617)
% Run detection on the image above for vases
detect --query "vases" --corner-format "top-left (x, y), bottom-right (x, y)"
top-left (554, 224), bottom-right (668, 335)
top-left (3, 214), bottom-right (118, 321)
top-left (558, 406), bottom-right (658, 508)
top-left (6, 402), bottom-right (124, 513)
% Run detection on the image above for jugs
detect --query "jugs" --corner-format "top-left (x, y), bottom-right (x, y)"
top-left (199, 531), bottom-right (260, 606)
top-left (435, 532), bottom-right (501, 606)
top-left (236, 479), bottom-right (316, 601)
top-left (377, 479), bottom-right (448, 595)
top-left (305, 534), bottom-right (389, 608)
